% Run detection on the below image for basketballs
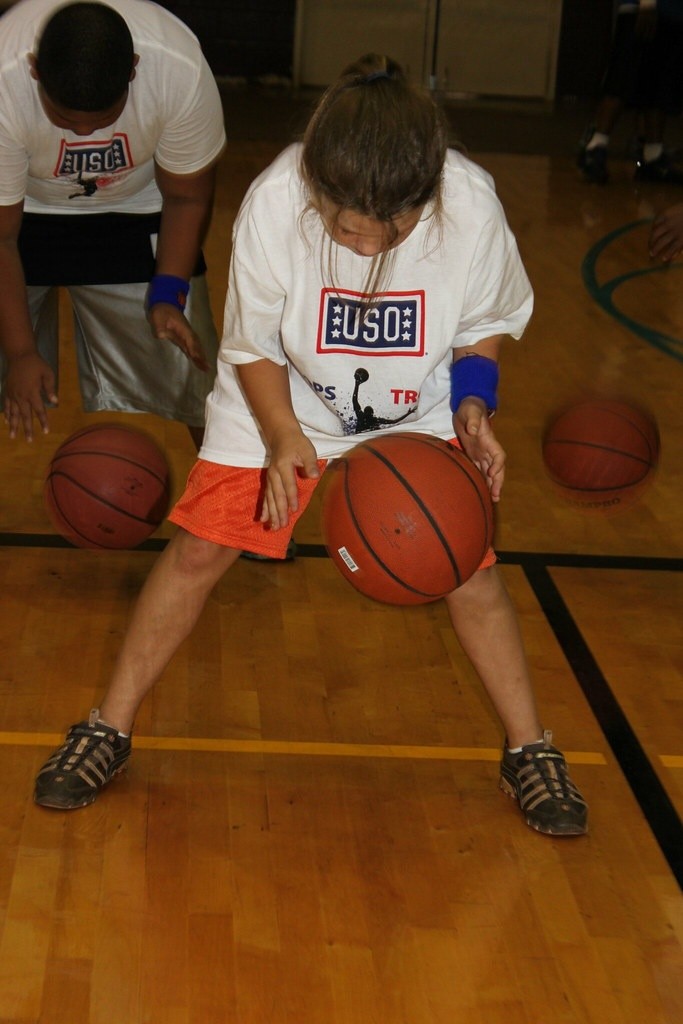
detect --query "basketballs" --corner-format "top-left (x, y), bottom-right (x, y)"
top-left (320, 431), bottom-right (497, 605)
top-left (540, 395), bottom-right (662, 515)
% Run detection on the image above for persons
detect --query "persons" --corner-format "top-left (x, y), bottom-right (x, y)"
top-left (34, 53), bottom-right (590, 838)
top-left (576, 2), bottom-right (682, 184)
top-left (2, 0), bottom-right (219, 442)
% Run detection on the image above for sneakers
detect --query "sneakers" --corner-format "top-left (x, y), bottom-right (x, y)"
top-left (499, 735), bottom-right (588, 834)
top-left (577, 143), bottom-right (612, 189)
top-left (633, 154), bottom-right (683, 184)
top-left (34, 708), bottom-right (132, 808)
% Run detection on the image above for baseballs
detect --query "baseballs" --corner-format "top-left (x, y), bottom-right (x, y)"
top-left (42, 422), bottom-right (175, 553)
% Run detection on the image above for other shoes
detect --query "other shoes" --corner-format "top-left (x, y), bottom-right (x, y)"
top-left (240, 535), bottom-right (296, 560)
